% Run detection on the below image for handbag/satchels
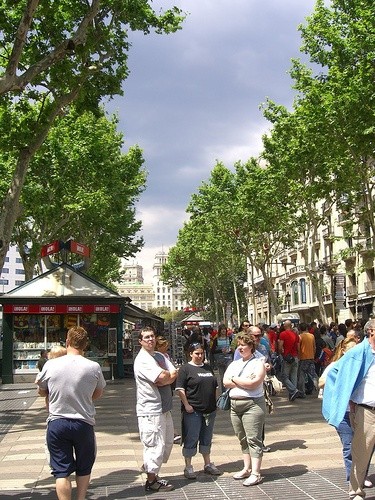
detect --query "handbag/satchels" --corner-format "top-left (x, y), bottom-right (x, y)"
top-left (216, 354), bottom-right (232, 365)
top-left (318, 348), bottom-right (332, 364)
top-left (285, 354), bottom-right (295, 364)
top-left (217, 391), bottom-right (231, 410)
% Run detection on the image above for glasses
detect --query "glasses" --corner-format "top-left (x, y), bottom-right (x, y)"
top-left (190, 342), bottom-right (204, 350)
top-left (244, 324), bottom-right (252, 328)
top-left (251, 334), bottom-right (262, 338)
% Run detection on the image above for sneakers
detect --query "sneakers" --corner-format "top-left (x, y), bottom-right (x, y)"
top-left (184, 465), bottom-right (197, 478)
top-left (145, 480), bottom-right (171, 492)
top-left (155, 476), bottom-right (175, 490)
top-left (204, 462), bottom-right (221, 474)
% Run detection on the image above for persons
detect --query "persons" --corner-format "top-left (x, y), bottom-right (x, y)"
top-left (133, 326), bottom-right (177, 491)
top-left (237, 319), bottom-right (365, 401)
top-left (319, 337), bottom-right (372, 487)
top-left (156, 336), bottom-right (177, 396)
top-left (37, 348), bottom-right (45, 371)
top-left (184, 324), bottom-right (240, 370)
top-left (45, 346), bottom-right (97, 468)
top-left (222, 334), bottom-right (269, 486)
top-left (176, 342), bottom-right (221, 478)
top-left (322, 320), bottom-right (375, 500)
top-left (35, 326), bottom-right (106, 500)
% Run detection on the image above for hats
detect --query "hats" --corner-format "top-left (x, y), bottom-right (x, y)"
top-left (269, 323), bottom-right (277, 328)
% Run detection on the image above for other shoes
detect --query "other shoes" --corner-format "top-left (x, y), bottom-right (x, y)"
top-left (233, 469), bottom-right (252, 479)
top-left (297, 392), bottom-right (306, 398)
top-left (290, 389), bottom-right (299, 401)
top-left (363, 477), bottom-right (372, 487)
top-left (243, 474), bottom-right (264, 486)
top-left (353, 495), bottom-right (363, 500)
top-left (312, 391), bottom-right (318, 398)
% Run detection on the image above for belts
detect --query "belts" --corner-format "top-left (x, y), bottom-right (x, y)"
top-left (360, 404), bottom-right (375, 410)
